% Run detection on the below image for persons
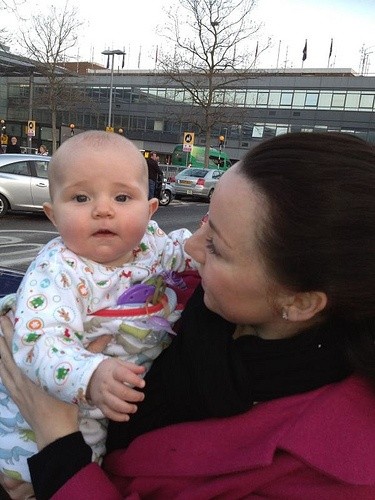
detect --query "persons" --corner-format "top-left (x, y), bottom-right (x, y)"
top-left (6, 136), bottom-right (21, 154)
top-left (0, 128), bottom-right (375, 500)
top-left (39, 145), bottom-right (49, 156)
top-left (147, 151), bottom-right (163, 201)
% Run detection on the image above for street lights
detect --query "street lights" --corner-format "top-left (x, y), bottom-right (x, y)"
top-left (100, 49), bottom-right (126, 133)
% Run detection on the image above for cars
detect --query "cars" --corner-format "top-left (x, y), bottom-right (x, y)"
top-left (167, 167), bottom-right (227, 203)
top-left (158, 184), bottom-right (176, 206)
top-left (0, 151), bottom-right (52, 219)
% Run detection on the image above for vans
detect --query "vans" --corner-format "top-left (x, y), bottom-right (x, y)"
top-left (170, 144), bottom-right (234, 173)
